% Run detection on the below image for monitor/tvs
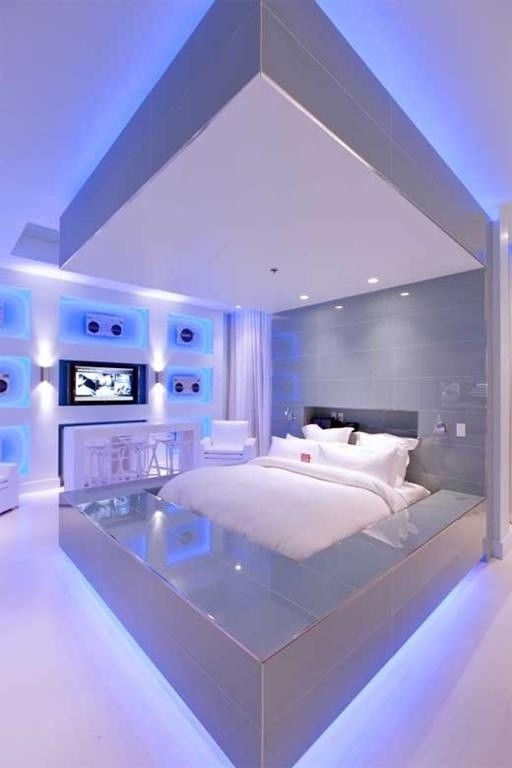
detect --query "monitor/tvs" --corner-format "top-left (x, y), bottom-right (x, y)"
top-left (58, 360), bottom-right (149, 406)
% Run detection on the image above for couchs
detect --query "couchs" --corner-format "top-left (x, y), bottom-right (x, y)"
top-left (0, 462), bottom-right (20, 515)
top-left (200, 419), bottom-right (257, 466)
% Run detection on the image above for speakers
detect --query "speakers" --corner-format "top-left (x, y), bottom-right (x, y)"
top-left (172, 377), bottom-right (201, 395)
top-left (176, 324), bottom-right (196, 346)
top-left (0, 372), bottom-right (10, 397)
top-left (85, 313), bottom-right (124, 337)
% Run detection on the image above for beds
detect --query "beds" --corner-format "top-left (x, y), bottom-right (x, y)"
top-left (157, 454), bottom-right (434, 556)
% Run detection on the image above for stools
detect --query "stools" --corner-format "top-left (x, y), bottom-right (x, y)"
top-left (90, 437), bottom-right (185, 485)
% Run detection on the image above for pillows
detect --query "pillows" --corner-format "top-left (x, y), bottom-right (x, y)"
top-left (303, 423), bottom-right (354, 443)
top-left (268, 433), bottom-right (400, 486)
top-left (354, 429), bottom-right (418, 486)
